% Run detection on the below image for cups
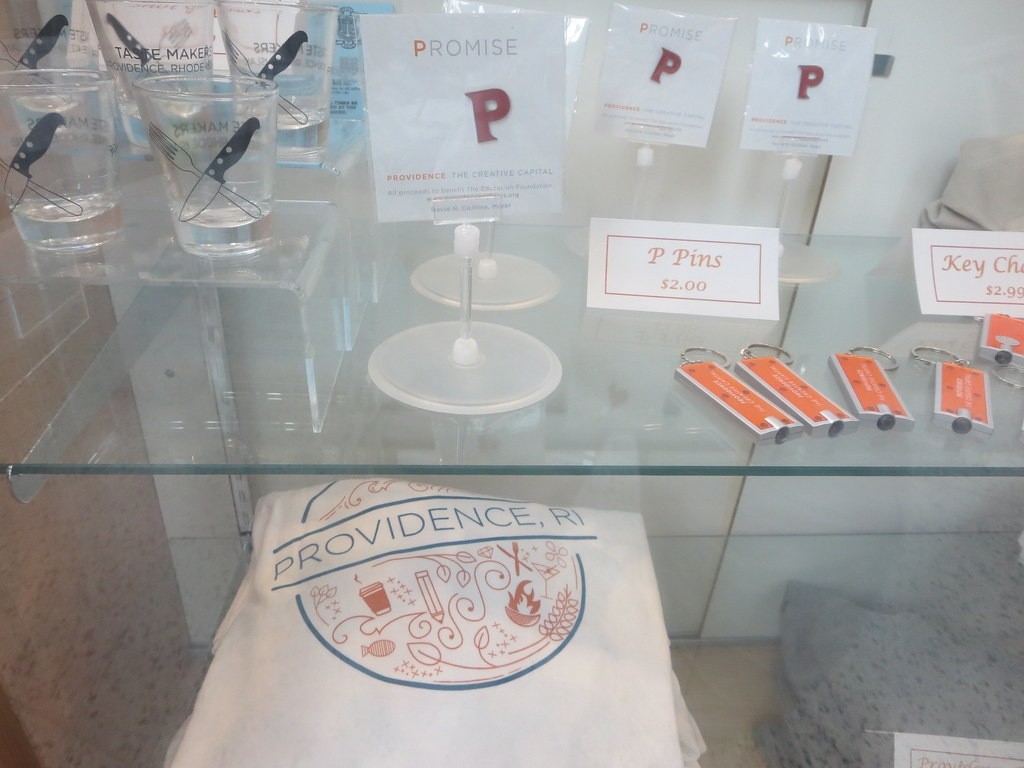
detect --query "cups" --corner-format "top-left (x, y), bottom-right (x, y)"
top-left (1, 1), bottom-right (100, 155)
top-left (131, 72), bottom-right (278, 259)
top-left (86, 0), bottom-right (217, 149)
top-left (214, 0), bottom-right (341, 163)
top-left (0, 68), bottom-right (122, 251)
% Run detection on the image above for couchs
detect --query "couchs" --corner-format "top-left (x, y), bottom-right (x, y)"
top-left (752, 509), bottom-right (1024, 768)
